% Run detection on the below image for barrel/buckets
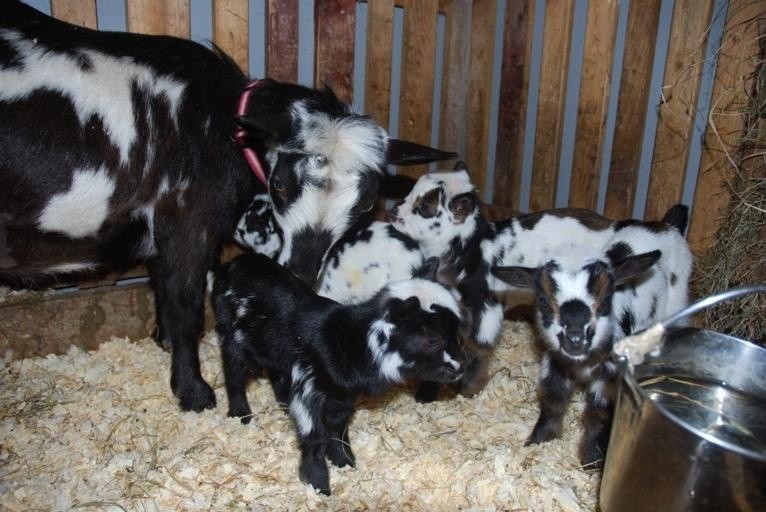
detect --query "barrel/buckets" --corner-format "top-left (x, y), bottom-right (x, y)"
top-left (595, 287), bottom-right (765, 512)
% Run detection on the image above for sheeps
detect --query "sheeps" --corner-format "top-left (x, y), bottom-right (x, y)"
top-left (207, 234), bottom-right (480, 497)
top-left (0, 0), bottom-right (459, 413)
top-left (232, 163), bottom-right (695, 473)
